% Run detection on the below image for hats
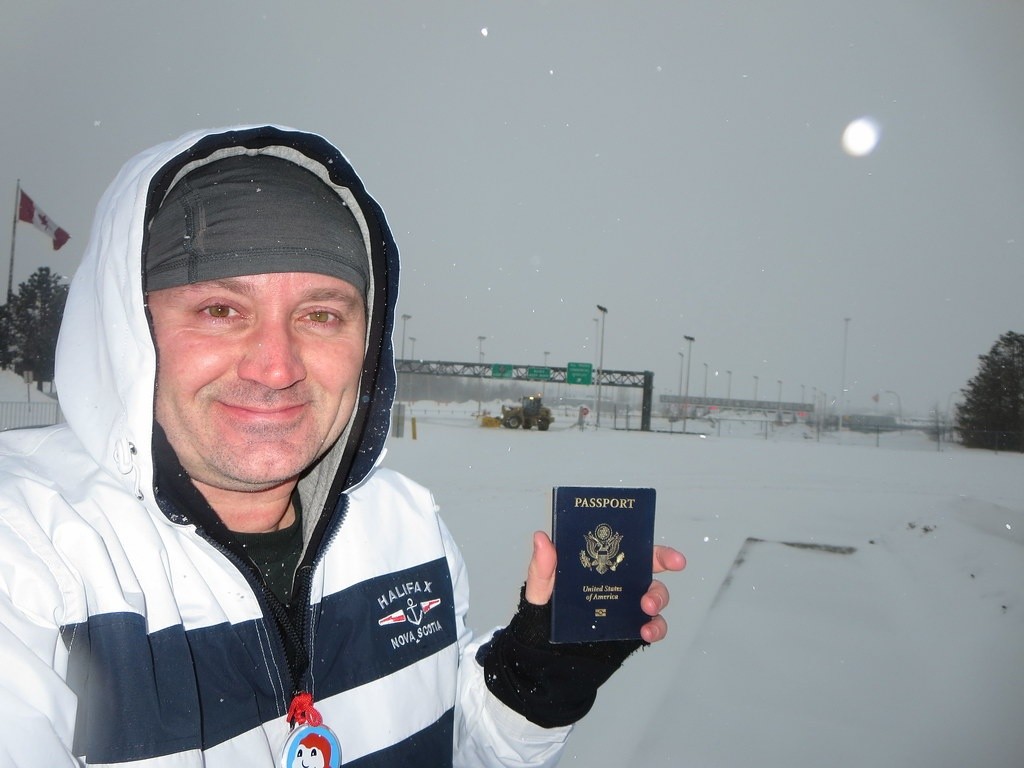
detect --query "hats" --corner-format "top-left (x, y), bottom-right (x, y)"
top-left (144, 155), bottom-right (370, 294)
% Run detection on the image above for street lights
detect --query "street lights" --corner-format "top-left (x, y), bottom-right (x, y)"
top-left (400, 314), bottom-right (411, 361)
top-left (684, 336), bottom-right (696, 432)
top-left (753, 375), bottom-right (759, 409)
top-left (885, 391), bottom-right (902, 439)
top-left (777, 379), bottom-right (784, 411)
top-left (543, 351), bottom-right (551, 399)
top-left (477, 336), bottom-right (486, 364)
top-left (838, 318), bottom-right (851, 432)
top-left (703, 362), bottom-right (708, 406)
top-left (593, 319), bottom-right (598, 406)
top-left (727, 371), bottom-right (732, 407)
top-left (812, 386), bottom-right (827, 433)
top-left (800, 383), bottom-right (805, 411)
top-left (409, 335), bottom-right (416, 360)
top-left (481, 352), bottom-right (485, 363)
top-left (596, 303), bottom-right (607, 428)
top-left (677, 352), bottom-right (683, 415)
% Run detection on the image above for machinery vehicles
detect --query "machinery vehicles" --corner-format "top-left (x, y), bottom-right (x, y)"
top-left (479, 393), bottom-right (555, 431)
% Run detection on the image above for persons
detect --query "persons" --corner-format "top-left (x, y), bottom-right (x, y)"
top-left (0, 125), bottom-right (685, 768)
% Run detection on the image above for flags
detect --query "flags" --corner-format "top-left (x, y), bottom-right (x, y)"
top-left (19, 189), bottom-right (70, 251)
